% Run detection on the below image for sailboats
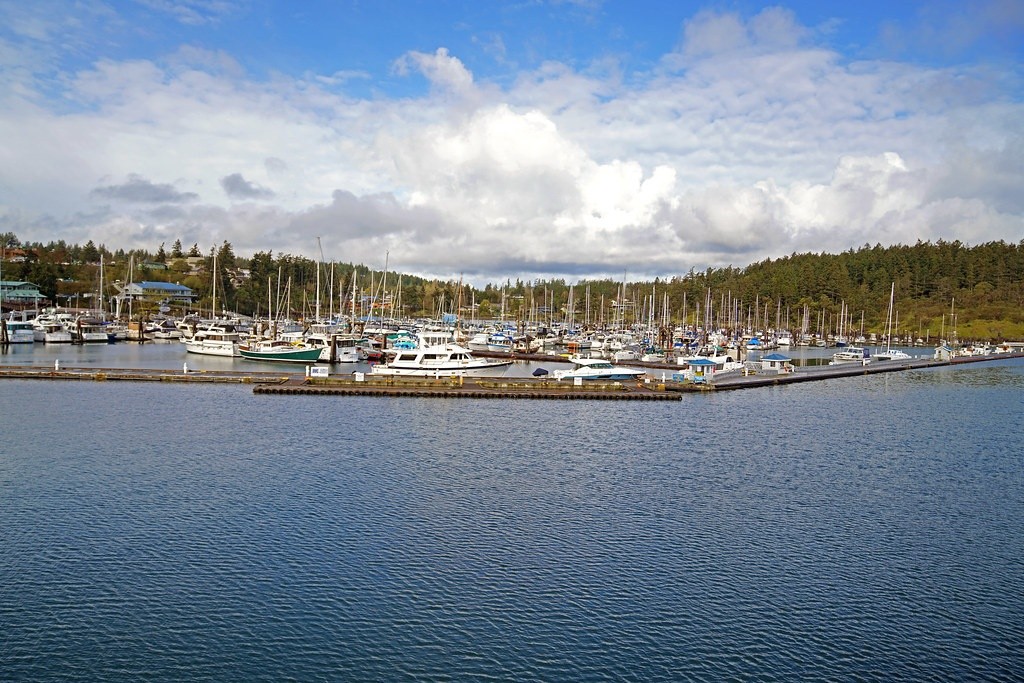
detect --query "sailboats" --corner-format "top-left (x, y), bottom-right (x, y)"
top-left (872, 282), bottom-right (913, 362)
top-left (1, 234), bottom-right (1016, 361)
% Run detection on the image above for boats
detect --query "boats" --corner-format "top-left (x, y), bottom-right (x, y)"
top-left (677, 347), bottom-right (747, 382)
top-left (829, 343), bottom-right (872, 366)
top-left (370, 291), bottom-right (517, 378)
top-left (551, 352), bottom-right (648, 380)
